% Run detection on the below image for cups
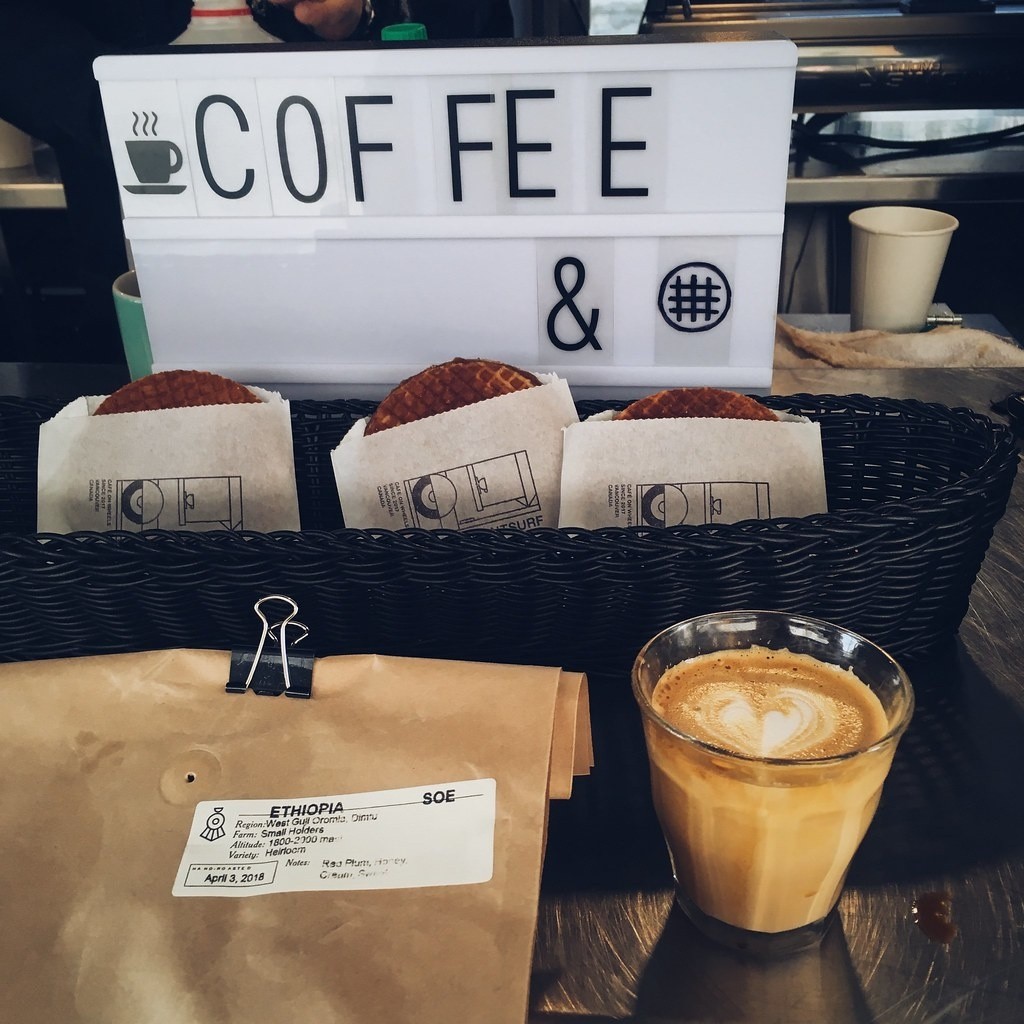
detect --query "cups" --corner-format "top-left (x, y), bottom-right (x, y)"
top-left (112, 269), bottom-right (152, 381)
top-left (631, 609), bottom-right (916, 961)
top-left (849, 206), bottom-right (959, 332)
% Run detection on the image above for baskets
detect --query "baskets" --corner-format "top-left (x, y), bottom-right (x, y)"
top-left (1, 392), bottom-right (1014, 679)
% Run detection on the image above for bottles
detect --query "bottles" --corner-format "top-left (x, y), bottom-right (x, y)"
top-left (169, 0), bottom-right (286, 44)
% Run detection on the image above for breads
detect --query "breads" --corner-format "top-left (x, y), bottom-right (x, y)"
top-left (364, 357), bottom-right (544, 433)
top-left (611, 386), bottom-right (781, 420)
top-left (90, 368), bottom-right (261, 416)
